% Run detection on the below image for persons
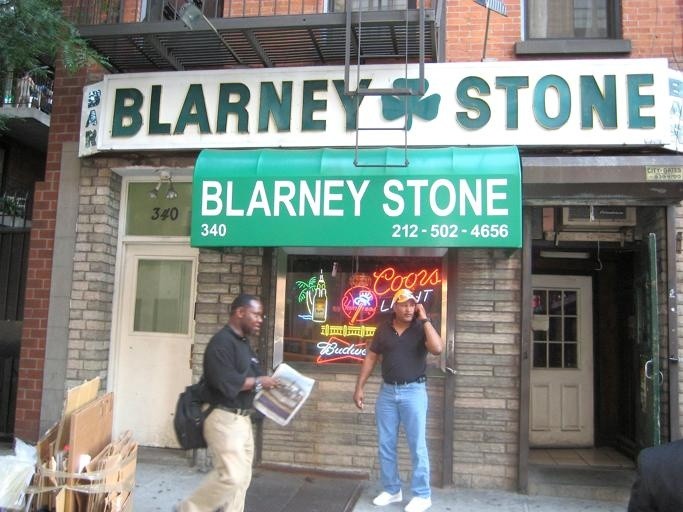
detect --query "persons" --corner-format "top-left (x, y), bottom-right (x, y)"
top-left (352, 288), bottom-right (442, 512)
top-left (627, 437), bottom-right (682, 512)
top-left (173, 295), bottom-right (282, 511)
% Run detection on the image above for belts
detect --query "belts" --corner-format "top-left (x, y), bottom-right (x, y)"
top-left (214, 403), bottom-right (251, 416)
top-left (383, 378), bottom-right (417, 385)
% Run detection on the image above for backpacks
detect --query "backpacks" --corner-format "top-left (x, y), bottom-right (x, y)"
top-left (175, 382), bottom-right (210, 449)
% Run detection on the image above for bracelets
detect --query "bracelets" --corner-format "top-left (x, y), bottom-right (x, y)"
top-left (419, 317), bottom-right (432, 322)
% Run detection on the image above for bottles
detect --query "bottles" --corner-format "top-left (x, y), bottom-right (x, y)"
top-left (49, 456), bottom-right (56, 482)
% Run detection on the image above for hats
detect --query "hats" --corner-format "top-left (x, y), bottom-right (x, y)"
top-left (395, 289), bottom-right (419, 304)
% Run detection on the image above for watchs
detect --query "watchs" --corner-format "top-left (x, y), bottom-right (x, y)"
top-left (254, 375), bottom-right (262, 393)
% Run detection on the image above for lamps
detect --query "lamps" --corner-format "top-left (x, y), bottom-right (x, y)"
top-left (146, 171), bottom-right (177, 200)
top-left (473, 0), bottom-right (508, 61)
top-left (174, 0), bottom-right (246, 66)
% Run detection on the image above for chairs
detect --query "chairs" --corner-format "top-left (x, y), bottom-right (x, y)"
top-left (14, 79), bottom-right (42, 111)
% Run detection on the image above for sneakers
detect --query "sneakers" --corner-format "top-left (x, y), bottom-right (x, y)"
top-left (372, 488), bottom-right (402, 506)
top-left (404, 496), bottom-right (432, 512)
